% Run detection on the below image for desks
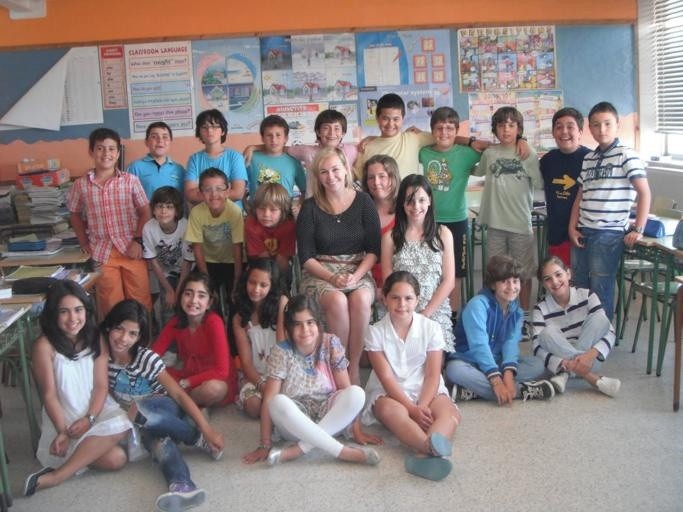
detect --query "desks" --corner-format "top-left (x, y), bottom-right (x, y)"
top-left (462, 206), bottom-right (487, 306)
top-left (0, 237), bottom-right (102, 512)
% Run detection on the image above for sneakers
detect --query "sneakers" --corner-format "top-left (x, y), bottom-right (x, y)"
top-left (194, 434), bottom-right (222, 459)
top-left (452, 384), bottom-right (479, 402)
top-left (517, 379), bottom-right (554, 401)
top-left (266, 442), bottom-right (299, 466)
top-left (344, 442), bottom-right (378, 464)
top-left (550, 370), bottom-right (568, 395)
top-left (593, 375), bottom-right (620, 397)
top-left (157, 481), bottom-right (204, 511)
top-left (24, 466), bottom-right (53, 496)
top-left (404, 453), bottom-right (450, 481)
top-left (428, 432), bottom-right (453, 458)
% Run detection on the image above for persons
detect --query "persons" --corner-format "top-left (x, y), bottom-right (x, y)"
top-left (232, 256), bottom-right (459, 481)
top-left (447, 255), bottom-right (621, 402)
top-left (69, 92), bottom-right (650, 386)
top-left (23, 271), bottom-right (235, 512)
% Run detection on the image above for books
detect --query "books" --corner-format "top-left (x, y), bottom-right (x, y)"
top-left (0, 180), bottom-right (90, 299)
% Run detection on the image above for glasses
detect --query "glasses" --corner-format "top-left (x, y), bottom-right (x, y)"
top-left (154, 202), bottom-right (173, 212)
top-left (201, 186), bottom-right (228, 196)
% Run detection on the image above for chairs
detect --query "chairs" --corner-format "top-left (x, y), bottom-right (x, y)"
top-left (531, 199), bottom-right (683, 414)
